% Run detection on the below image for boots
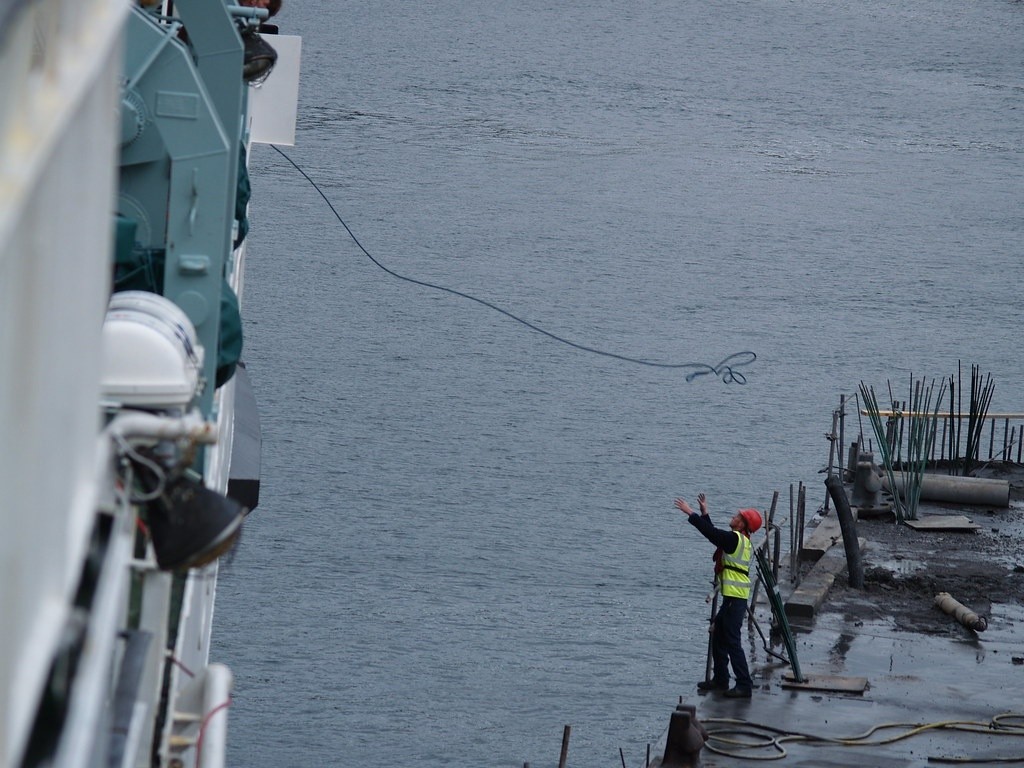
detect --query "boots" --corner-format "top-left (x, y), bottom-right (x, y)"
top-left (723, 679), bottom-right (752, 700)
top-left (698, 674), bottom-right (730, 691)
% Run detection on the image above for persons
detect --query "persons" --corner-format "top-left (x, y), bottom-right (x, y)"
top-left (674, 493), bottom-right (763, 697)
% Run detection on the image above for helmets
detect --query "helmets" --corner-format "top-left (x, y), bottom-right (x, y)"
top-left (738, 508), bottom-right (762, 533)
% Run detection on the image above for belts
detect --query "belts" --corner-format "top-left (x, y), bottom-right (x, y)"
top-left (723, 563), bottom-right (750, 576)
